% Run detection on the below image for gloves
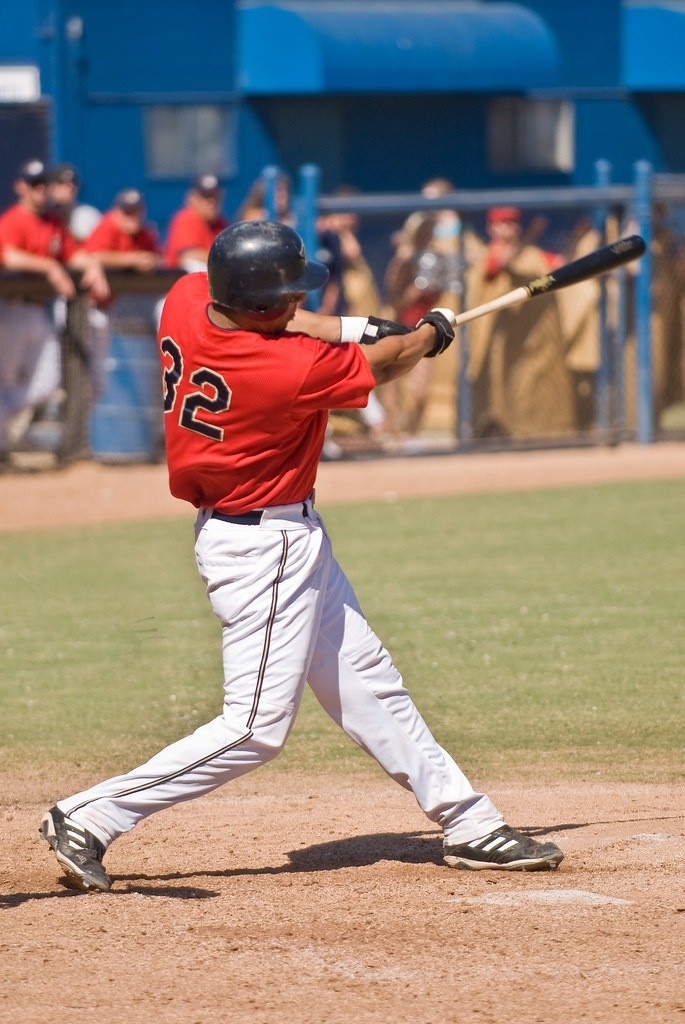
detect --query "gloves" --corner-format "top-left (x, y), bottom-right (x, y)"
top-left (410, 311), bottom-right (455, 358)
top-left (360, 316), bottom-right (414, 345)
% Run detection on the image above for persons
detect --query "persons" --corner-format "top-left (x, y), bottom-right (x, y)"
top-left (0, 159), bottom-right (684, 469)
top-left (39, 219), bottom-right (563, 892)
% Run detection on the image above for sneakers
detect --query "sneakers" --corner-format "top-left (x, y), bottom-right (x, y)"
top-left (38, 805), bottom-right (111, 890)
top-left (443, 824), bottom-right (564, 870)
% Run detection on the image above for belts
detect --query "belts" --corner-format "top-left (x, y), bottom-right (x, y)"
top-left (202, 489), bottom-right (316, 523)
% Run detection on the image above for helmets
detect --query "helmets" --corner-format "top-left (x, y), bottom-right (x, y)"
top-left (208, 219), bottom-right (330, 313)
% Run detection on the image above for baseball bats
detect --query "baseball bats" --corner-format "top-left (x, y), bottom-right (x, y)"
top-left (452, 234), bottom-right (646, 325)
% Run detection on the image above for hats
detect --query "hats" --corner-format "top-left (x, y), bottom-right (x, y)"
top-left (114, 188), bottom-right (144, 209)
top-left (47, 163), bottom-right (83, 188)
top-left (486, 207), bottom-right (520, 222)
top-left (21, 160), bottom-right (53, 182)
top-left (194, 176), bottom-right (223, 195)
